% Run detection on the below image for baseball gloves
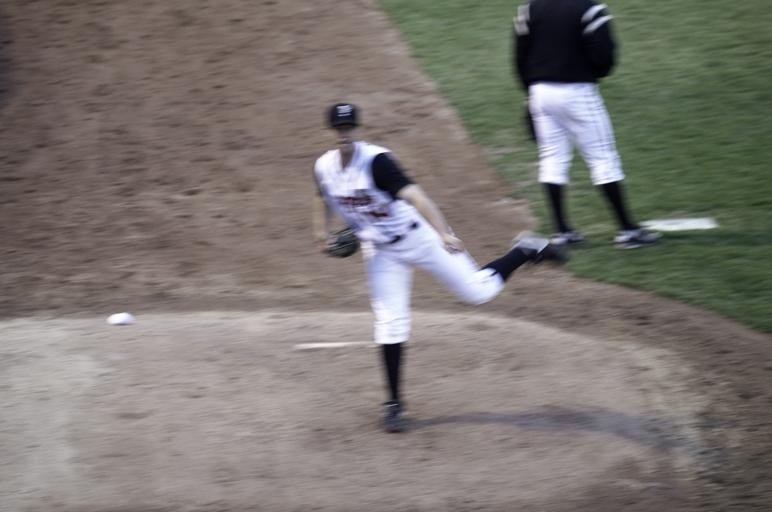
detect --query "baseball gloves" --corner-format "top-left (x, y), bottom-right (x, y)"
top-left (319, 223), bottom-right (358, 258)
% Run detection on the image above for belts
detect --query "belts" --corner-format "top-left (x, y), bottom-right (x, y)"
top-left (390, 223), bottom-right (418, 245)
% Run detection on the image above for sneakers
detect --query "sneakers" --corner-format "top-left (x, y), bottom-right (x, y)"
top-left (613, 228), bottom-right (664, 250)
top-left (381, 398), bottom-right (407, 432)
top-left (514, 230), bottom-right (584, 265)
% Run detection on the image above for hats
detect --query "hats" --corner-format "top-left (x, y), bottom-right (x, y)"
top-left (328, 104), bottom-right (360, 127)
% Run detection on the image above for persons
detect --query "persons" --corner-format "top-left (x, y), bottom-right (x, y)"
top-left (311, 101), bottom-right (571, 434)
top-left (509, 0), bottom-right (665, 253)
